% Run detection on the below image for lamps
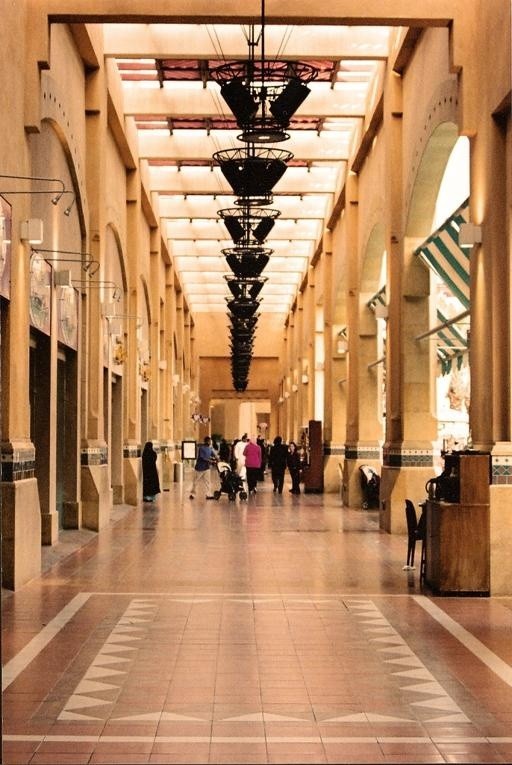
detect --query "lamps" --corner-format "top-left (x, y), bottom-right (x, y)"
top-left (0, 176), bottom-right (122, 302)
top-left (213, 8), bottom-right (317, 389)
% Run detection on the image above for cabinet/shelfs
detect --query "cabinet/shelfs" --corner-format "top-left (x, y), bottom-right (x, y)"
top-left (304, 420), bottom-right (322, 493)
top-left (424, 453), bottom-right (490, 592)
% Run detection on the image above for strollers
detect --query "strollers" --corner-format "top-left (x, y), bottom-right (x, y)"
top-left (208, 456), bottom-right (251, 502)
top-left (358, 462), bottom-right (383, 512)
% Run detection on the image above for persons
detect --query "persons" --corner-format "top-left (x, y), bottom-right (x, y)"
top-left (142, 442), bottom-right (161, 502)
top-left (189, 433), bottom-right (303, 500)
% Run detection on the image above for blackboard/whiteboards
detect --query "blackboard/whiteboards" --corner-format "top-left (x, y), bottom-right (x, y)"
top-left (182, 441), bottom-right (197, 460)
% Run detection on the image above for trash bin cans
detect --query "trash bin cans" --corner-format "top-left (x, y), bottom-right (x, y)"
top-left (174, 462), bottom-right (184, 483)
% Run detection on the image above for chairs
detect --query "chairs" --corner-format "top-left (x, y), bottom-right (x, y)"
top-left (404, 499), bottom-right (423, 566)
